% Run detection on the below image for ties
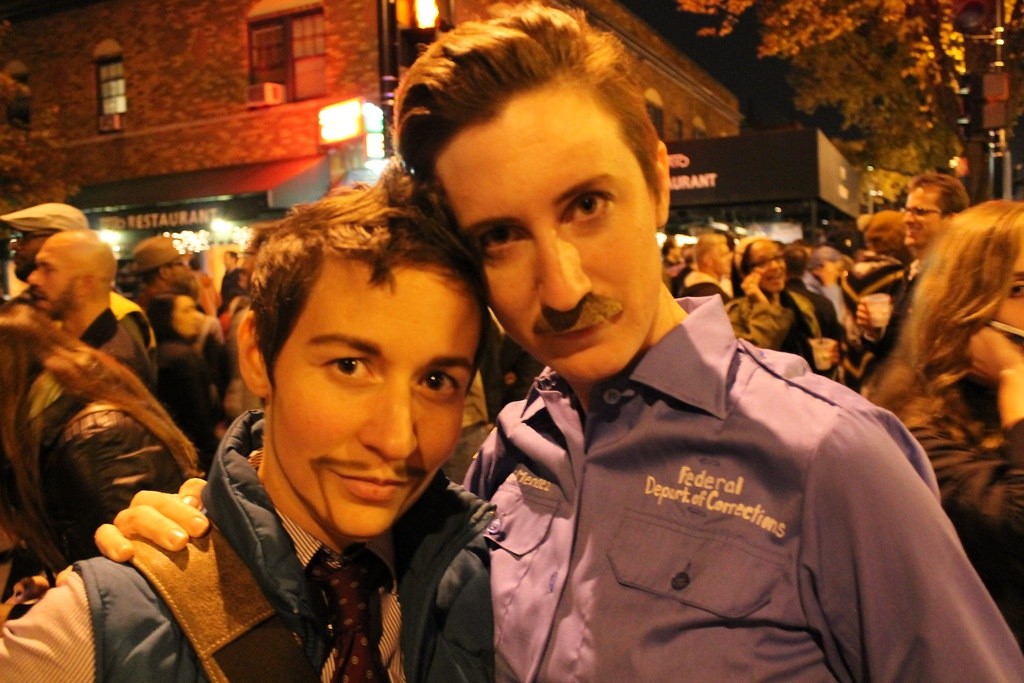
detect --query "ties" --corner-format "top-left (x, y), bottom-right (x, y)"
top-left (308, 555), bottom-right (383, 683)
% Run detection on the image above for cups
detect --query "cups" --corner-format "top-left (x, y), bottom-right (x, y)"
top-left (866, 293), bottom-right (892, 328)
top-left (811, 338), bottom-right (837, 370)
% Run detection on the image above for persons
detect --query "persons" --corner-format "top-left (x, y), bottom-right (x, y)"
top-left (0, 175), bottom-right (1023, 654)
top-left (93, 4), bottom-right (1024, 683)
top-left (0, 164), bottom-right (494, 683)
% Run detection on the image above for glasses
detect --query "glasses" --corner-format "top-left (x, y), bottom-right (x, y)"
top-left (13, 232), bottom-right (52, 244)
top-left (745, 251), bottom-right (785, 270)
top-left (900, 206), bottom-right (944, 217)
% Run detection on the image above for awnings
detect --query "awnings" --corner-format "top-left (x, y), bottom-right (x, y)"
top-left (70, 155), bottom-right (331, 231)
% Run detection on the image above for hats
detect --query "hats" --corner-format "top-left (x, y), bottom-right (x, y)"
top-left (0, 203), bottom-right (89, 235)
top-left (127, 237), bottom-right (183, 273)
top-left (734, 234), bottom-right (781, 272)
